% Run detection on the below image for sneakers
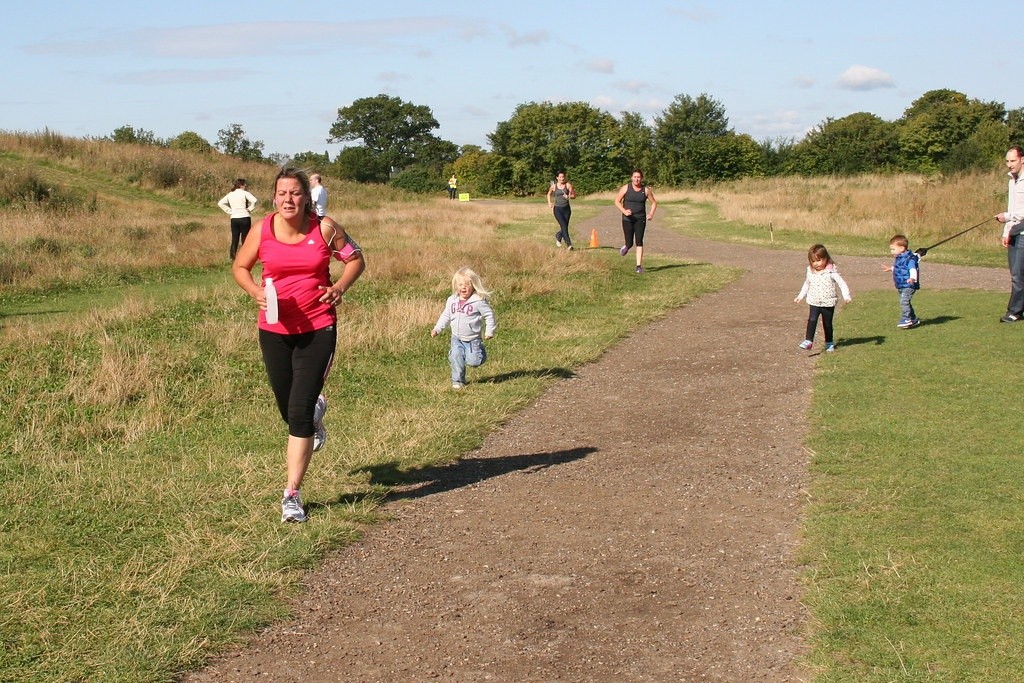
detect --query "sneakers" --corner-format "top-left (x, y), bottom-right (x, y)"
top-left (799, 340), bottom-right (812, 350)
top-left (825, 344), bottom-right (834, 351)
top-left (281, 489), bottom-right (307, 523)
top-left (897, 319), bottom-right (913, 327)
top-left (313, 394), bottom-right (327, 452)
top-left (905, 320), bottom-right (919, 328)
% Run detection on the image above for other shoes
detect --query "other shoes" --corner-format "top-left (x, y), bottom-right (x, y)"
top-left (452, 384), bottom-right (460, 389)
top-left (1000, 313), bottom-right (1019, 322)
top-left (568, 246), bottom-right (573, 251)
top-left (636, 267), bottom-right (643, 273)
top-left (620, 245), bottom-right (629, 256)
top-left (557, 240), bottom-right (562, 247)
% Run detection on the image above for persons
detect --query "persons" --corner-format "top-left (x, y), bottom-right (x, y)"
top-left (309, 174), bottom-right (327, 217)
top-left (448, 174), bottom-right (458, 200)
top-left (614, 169), bottom-right (657, 273)
top-left (794, 244), bottom-right (851, 352)
top-left (431, 267), bottom-right (494, 389)
top-left (995, 146), bottom-right (1024, 322)
top-left (218, 179), bottom-right (258, 260)
top-left (234, 167), bottom-right (366, 524)
top-left (881, 236), bottom-right (920, 328)
top-left (547, 170), bottom-right (575, 251)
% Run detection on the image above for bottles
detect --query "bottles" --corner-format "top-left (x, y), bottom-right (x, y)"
top-left (264, 278), bottom-right (279, 324)
